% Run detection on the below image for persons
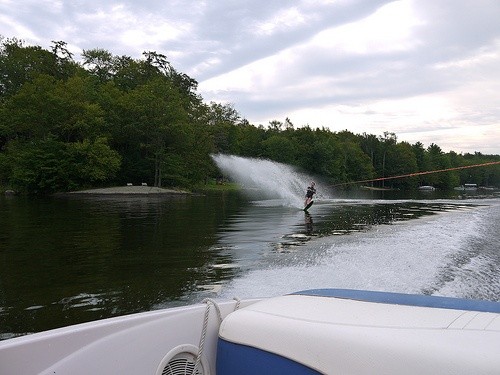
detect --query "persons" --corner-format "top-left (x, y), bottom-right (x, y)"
top-left (304, 181), bottom-right (316, 207)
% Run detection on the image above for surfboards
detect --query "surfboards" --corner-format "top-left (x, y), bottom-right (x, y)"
top-left (304, 201), bottom-right (313, 210)
